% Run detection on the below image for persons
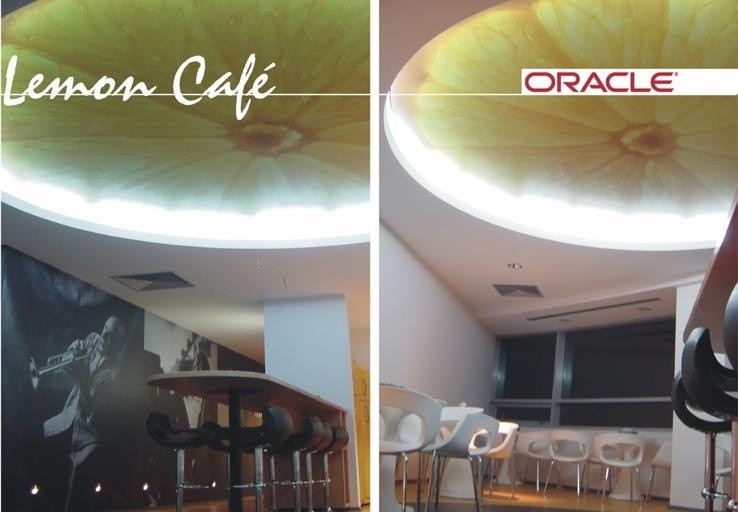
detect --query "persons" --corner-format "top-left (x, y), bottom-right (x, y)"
top-left (42, 315), bottom-right (127, 512)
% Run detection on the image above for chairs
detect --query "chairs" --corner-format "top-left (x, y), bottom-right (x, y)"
top-left (145, 407), bottom-right (350, 512)
top-left (673, 281), bottom-right (738, 510)
top-left (379, 385), bottom-right (671, 511)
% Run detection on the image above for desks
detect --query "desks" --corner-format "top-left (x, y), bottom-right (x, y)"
top-left (148, 370), bottom-right (348, 512)
